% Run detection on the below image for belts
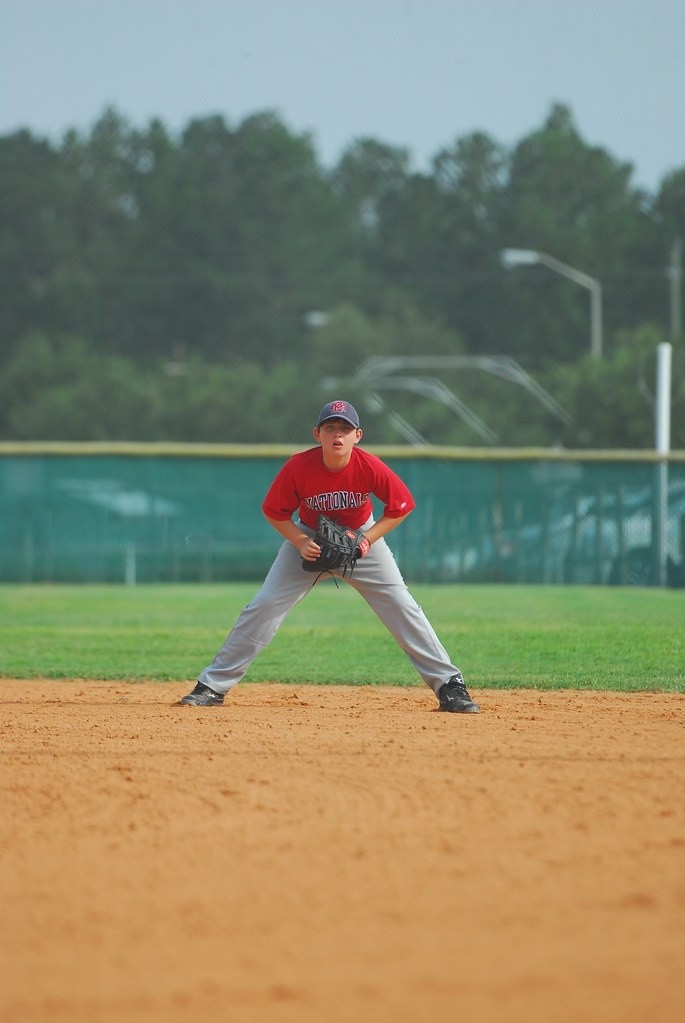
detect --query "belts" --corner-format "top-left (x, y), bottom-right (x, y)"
top-left (297, 517), bottom-right (309, 528)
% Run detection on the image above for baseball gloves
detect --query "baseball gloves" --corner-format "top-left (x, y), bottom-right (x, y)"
top-left (300, 513), bottom-right (372, 590)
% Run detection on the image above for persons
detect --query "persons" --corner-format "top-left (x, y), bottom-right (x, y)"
top-left (180, 400), bottom-right (481, 713)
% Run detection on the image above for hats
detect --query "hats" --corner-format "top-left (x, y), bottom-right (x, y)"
top-left (318, 400), bottom-right (360, 429)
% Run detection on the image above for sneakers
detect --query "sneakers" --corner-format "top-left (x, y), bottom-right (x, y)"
top-left (180, 681), bottom-right (224, 707)
top-left (440, 675), bottom-right (479, 713)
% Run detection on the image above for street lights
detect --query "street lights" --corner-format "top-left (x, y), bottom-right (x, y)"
top-left (504, 248), bottom-right (601, 363)
